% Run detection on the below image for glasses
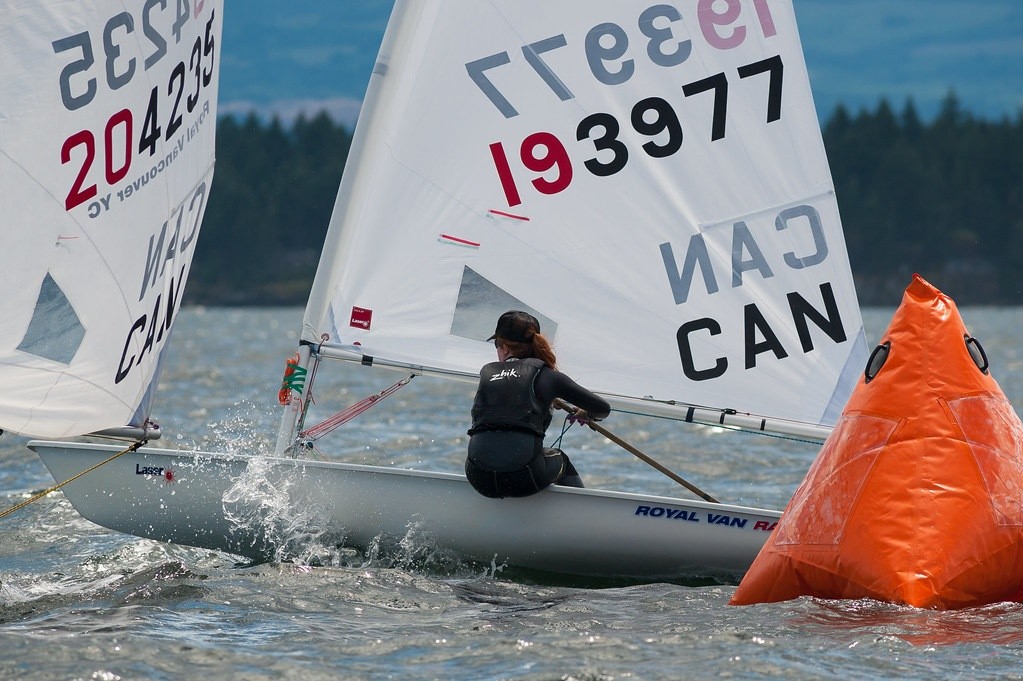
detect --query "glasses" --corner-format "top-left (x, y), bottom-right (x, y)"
top-left (495, 339), bottom-right (499, 348)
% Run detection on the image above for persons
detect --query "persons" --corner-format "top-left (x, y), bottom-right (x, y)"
top-left (464, 310), bottom-right (611, 499)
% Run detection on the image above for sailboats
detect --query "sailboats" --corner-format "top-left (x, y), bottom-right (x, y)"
top-left (1, 1), bottom-right (879, 587)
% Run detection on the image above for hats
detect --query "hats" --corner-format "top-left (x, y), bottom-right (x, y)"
top-left (486, 310), bottom-right (540, 344)
